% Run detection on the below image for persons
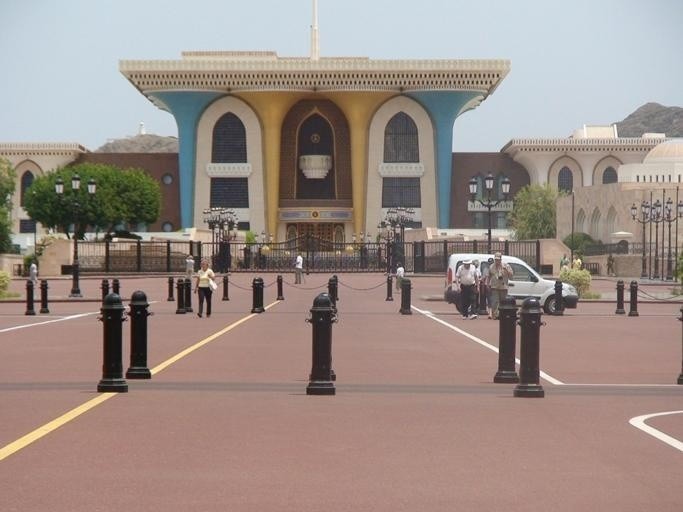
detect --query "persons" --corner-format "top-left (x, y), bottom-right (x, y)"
top-left (472, 259), bottom-right (485, 315)
top-left (182, 255), bottom-right (195, 283)
top-left (194, 257), bottom-right (215, 318)
top-left (390, 261), bottom-right (404, 293)
top-left (486, 251), bottom-right (514, 321)
top-left (606, 253), bottom-right (616, 276)
top-left (572, 254), bottom-right (582, 271)
top-left (456, 258), bottom-right (479, 320)
top-left (560, 253), bottom-right (570, 271)
top-left (481, 257), bottom-right (496, 319)
top-left (292, 252), bottom-right (303, 284)
top-left (29, 259), bottom-right (40, 285)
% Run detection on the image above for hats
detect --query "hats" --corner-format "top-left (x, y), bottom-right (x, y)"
top-left (495, 252), bottom-right (501, 259)
top-left (462, 258), bottom-right (472, 264)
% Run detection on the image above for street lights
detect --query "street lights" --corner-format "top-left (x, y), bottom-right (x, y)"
top-left (56, 170), bottom-right (98, 299)
top-left (199, 202), bottom-right (274, 275)
top-left (629, 198), bottom-right (683, 283)
top-left (468, 171), bottom-right (511, 255)
top-left (352, 205), bottom-right (418, 272)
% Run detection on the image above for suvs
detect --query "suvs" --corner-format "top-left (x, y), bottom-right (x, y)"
top-left (442, 252), bottom-right (578, 316)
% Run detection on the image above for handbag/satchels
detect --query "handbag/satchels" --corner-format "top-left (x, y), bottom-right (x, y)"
top-left (209, 277), bottom-right (217, 291)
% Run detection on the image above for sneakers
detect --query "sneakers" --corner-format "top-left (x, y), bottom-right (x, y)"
top-left (198, 313), bottom-right (202, 317)
top-left (470, 315), bottom-right (477, 319)
top-left (462, 316), bottom-right (467, 320)
top-left (488, 315), bottom-right (498, 319)
